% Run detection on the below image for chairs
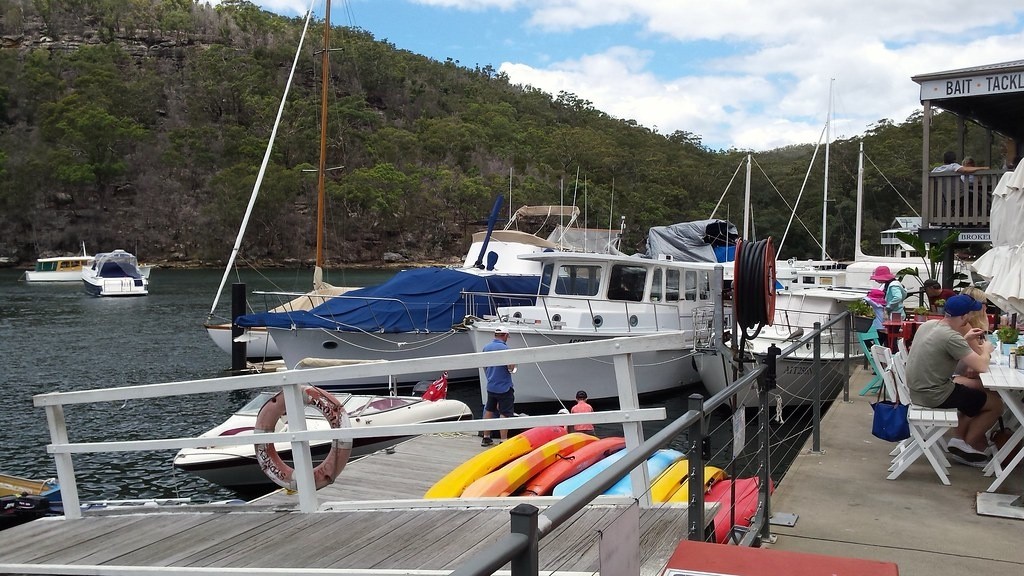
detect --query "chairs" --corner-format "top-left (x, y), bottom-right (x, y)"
top-left (857, 325), bottom-right (958, 486)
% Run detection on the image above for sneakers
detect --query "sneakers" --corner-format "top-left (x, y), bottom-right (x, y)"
top-left (947, 437), bottom-right (988, 462)
top-left (952, 453), bottom-right (990, 467)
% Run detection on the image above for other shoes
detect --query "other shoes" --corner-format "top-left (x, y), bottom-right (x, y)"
top-left (481, 436), bottom-right (493, 446)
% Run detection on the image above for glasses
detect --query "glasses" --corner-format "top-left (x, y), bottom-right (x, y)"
top-left (960, 293), bottom-right (976, 316)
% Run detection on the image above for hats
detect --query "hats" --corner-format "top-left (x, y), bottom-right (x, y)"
top-left (494, 326), bottom-right (511, 338)
top-left (944, 295), bottom-right (982, 317)
top-left (924, 278), bottom-right (938, 287)
top-left (866, 288), bottom-right (887, 306)
top-left (870, 265), bottom-right (896, 281)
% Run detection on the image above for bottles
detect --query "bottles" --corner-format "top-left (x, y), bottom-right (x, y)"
top-left (978, 332), bottom-right (986, 345)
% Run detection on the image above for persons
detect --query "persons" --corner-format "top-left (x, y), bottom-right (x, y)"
top-left (961, 156), bottom-right (990, 197)
top-left (571, 390), bottom-right (597, 436)
top-left (905, 294), bottom-right (1003, 468)
top-left (864, 290), bottom-right (889, 375)
top-left (480, 325), bottom-right (515, 446)
top-left (869, 266), bottom-right (907, 321)
top-left (953, 286), bottom-right (989, 378)
top-left (924, 279), bottom-right (958, 312)
top-left (932, 151), bottom-right (982, 228)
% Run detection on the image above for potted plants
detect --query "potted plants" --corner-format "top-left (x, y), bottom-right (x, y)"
top-left (849, 300), bottom-right (874, 332)
top-left (913, 306), bottom-right (926, 321)
top-left (935, 299), bottom-right (945, 314)
top-left (1001, 329), bottom-right (1018, 355)
top-left (1015, 346), bottom-right (1024, 370)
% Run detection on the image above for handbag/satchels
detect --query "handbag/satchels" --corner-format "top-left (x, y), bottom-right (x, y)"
top-left (869, 371), bottom-right (911, 441)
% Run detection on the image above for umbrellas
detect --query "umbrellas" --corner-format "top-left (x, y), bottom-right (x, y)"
top-left (984, 153), bottom-right (1024, 318)
top-left (971, 171), bottom-right (1013, 283)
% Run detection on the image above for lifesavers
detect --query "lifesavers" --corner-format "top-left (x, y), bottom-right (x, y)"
top-left (254, 384), bottom-right (353, 492)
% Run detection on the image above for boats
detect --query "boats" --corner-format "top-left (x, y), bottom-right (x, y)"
top-left (203, 2), bottom-right (976, 437)
top-left (81, 249), bottom-right (149, 297)
top-left (172, 356), bottom-right (474, 502)
top-left (26, 240), bottom-right (157, 282)
top-left (0, 472), bottom-right (63, 526)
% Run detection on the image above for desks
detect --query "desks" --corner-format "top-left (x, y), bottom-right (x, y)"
top-left (883, 320), bottom-right (921, 350)
top-left (978, 335), bottom-right (1024, 493)
